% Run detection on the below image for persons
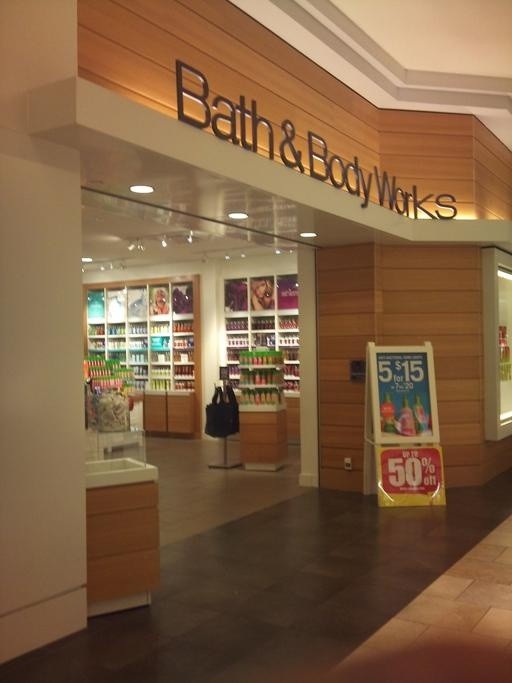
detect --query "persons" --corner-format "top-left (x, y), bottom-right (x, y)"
top-left (149, 287), bottom-right (170, 313)
top-left (250, 278), bottom-right (274, 310)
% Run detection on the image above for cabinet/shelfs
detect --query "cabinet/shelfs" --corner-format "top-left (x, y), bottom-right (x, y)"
top-left (82, 274), bottom-right (203, 440)
top-left (238, 364), bottom-right (287, 471)
top-left (223, 274), bottom-right (300, 445)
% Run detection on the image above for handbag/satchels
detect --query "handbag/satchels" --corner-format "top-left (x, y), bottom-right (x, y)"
top-left (205, 403), bottom-right (239, 437)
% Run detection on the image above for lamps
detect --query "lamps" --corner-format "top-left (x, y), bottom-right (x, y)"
top-left (224, 245), bottom-right (282, 260)
top-left (124, 229), bottom-right (201, 251)
